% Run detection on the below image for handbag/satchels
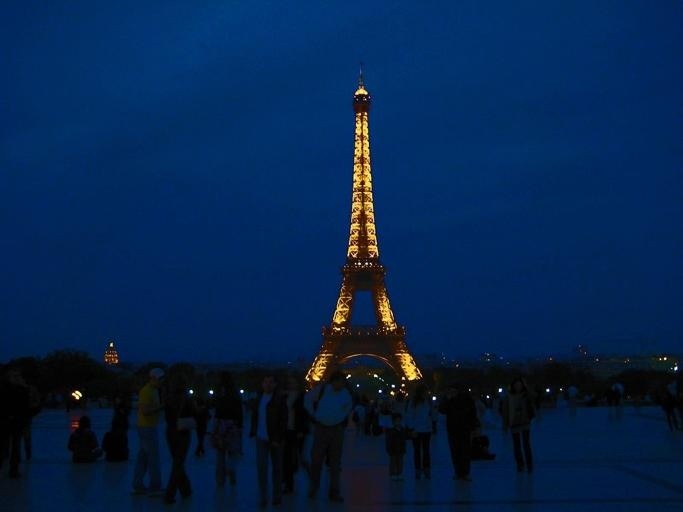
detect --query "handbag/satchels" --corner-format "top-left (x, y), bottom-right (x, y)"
top-left (313, 400), bottom-right (320, 412)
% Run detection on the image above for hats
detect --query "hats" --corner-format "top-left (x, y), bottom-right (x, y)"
top-left (150, 367), bottom-right (164, 380)
